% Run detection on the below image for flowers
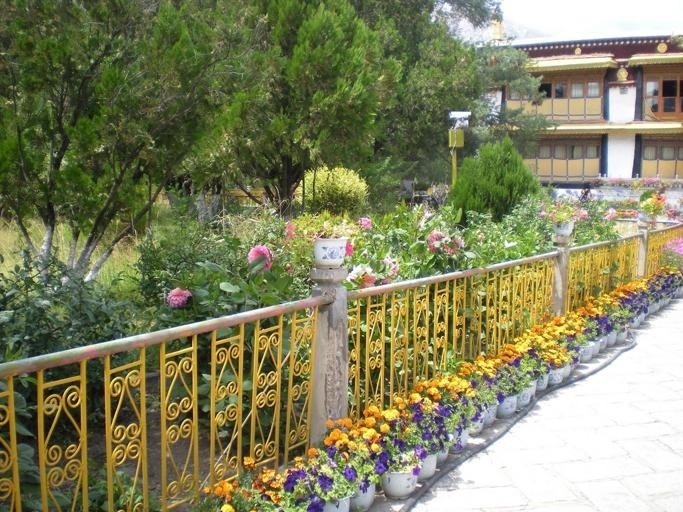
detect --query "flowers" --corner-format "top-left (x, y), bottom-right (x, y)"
top-left (538, 199), bottom-right (589, 223)
top-left (287, 210), bottom-right (371, 241)
top-left (465, 296), bottom-right (593, 438)
top-left (197, 375), bottom-right (481, 511)
top-left (586, 263), bottom-right (682, 359)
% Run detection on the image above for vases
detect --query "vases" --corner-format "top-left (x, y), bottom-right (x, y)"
top-left (314, 238), bottom-right (347, 270)
top-left (550, 220), bottom-right (575, 237)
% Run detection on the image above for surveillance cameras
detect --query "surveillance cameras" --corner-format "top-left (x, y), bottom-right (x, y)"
top-left (449, 110), bottom-right (473, 120)
